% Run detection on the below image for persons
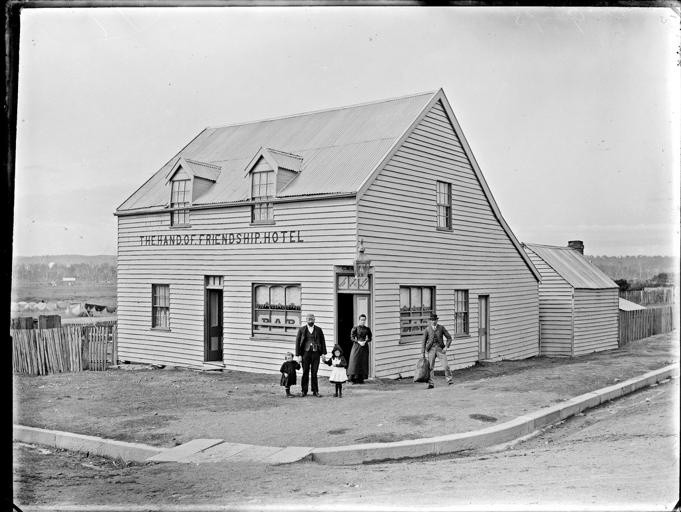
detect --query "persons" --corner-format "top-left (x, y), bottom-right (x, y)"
top-left (278, 352), bottom-right (301, 398)
top-left (420, 314), bottom-right (454, 389)
top-left (323, 344), bottom-right (348, 398)
top-left (294, 313), bottom-right (328, 398)
top-left (346, 313), bottom-right (372, 386)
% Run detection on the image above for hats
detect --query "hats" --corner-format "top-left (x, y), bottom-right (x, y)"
top-left (428, 313), bottom-right (440, 320)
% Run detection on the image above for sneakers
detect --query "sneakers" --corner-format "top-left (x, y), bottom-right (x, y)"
top-left (300, 391), bottom-right (308, 397)
top-left (313, 391), bottom-right (322, 397)
top-left (429, 379), bottom-right (455, 388)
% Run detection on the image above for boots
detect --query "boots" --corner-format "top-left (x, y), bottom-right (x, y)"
top-left (286, 389), bottom-right (295, 398)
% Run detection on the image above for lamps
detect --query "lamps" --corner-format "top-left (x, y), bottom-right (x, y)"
top-left (352, 237), bottom-right (370, 279)
